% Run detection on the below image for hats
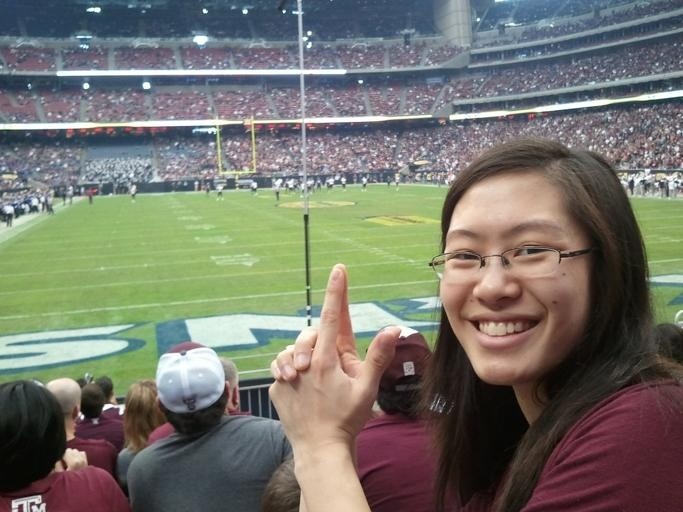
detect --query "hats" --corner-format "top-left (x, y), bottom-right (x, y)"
top-left (378, 324), bottom-right (432, 394)
top-left (155, 340), bottom-right (225, 416)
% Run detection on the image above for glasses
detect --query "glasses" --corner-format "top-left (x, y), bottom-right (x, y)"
top-left (428, 246), bottom-right (596, 279)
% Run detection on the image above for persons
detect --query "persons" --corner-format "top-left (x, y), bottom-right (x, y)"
top-left (1, 0), bottom-right (683, 226)
top-left (0, 138), bottom-right (683, 512)
